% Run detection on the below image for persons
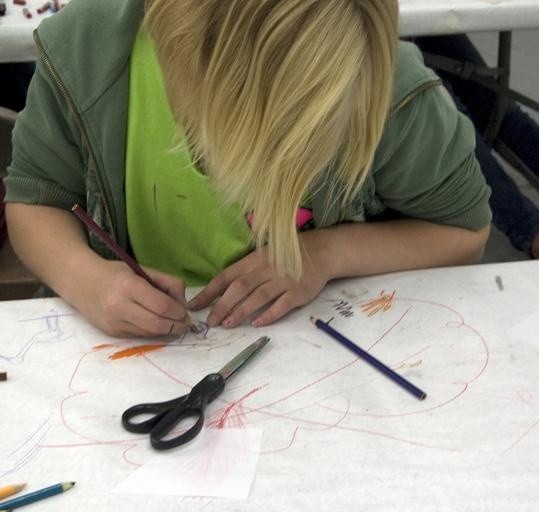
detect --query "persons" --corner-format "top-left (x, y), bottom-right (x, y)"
top-left (3, 0), bottom-right (492, 338)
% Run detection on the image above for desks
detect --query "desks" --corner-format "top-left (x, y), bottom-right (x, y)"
top-left (0, 1), bottom-right (539, 148)
top-left (0, 259), bottom-right (539, 512)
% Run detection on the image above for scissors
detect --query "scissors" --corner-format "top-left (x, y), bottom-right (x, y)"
top-left (122, 336), bottom-right (271, 450)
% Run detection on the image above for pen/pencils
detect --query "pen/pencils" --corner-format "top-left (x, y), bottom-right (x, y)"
top-left (310, 315), bottom-right (426, 400)
top-left (70, 204), bottom-right (199, 334)
top-left (0, 481), bottom-right (75, 510)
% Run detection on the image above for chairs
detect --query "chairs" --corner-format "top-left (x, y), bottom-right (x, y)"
top-left (0, 108), bottom-right (70, 300)
